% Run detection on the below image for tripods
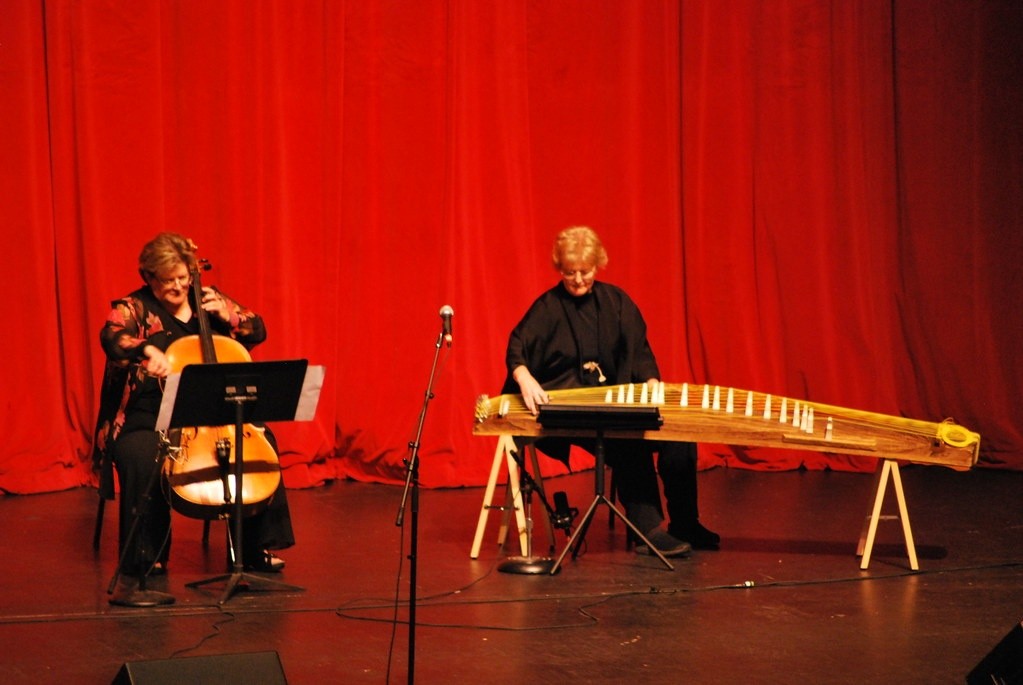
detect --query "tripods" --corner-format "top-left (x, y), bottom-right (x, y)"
top-left (531, 401), bottom-right (674, 577)
top-left (170, 358), bottom-right (310, 614)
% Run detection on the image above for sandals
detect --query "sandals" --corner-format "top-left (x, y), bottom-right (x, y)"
top-left (140, 564), bottom-right (168, 592)
top-left (227, 545), bottom-right (284, 574)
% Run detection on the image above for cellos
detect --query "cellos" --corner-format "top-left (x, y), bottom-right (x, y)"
top-left (158, 236), bottom-right (309, 607)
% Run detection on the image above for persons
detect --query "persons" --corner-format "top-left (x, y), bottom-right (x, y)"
top-left (100, 232), bottom-right (296, 579)
top-left (505, 226), bottom-right (721, 556)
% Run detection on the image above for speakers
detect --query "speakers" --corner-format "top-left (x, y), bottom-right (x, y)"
top-left (105, 649), bottom-right (287, 685)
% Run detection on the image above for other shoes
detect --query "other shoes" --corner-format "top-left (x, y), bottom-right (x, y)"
top-left (627, 529), bottom-right (691, 556)
top-left (667, 521), bottom-right (720, 545)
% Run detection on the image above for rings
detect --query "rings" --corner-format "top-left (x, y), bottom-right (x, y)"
top-left (153, 366), bottom-right (159, 370)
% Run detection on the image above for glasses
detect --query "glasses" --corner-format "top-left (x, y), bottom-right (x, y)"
top-left (560, 267), bottom-right (593, 279)
top-left (151, 273), bottom-right (193, 286)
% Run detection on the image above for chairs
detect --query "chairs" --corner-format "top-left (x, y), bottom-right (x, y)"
top-left (91, 356), bottom-right (211, 552)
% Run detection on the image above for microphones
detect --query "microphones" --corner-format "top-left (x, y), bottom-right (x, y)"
top-left (439, 304), bottom-right (454, 344)
